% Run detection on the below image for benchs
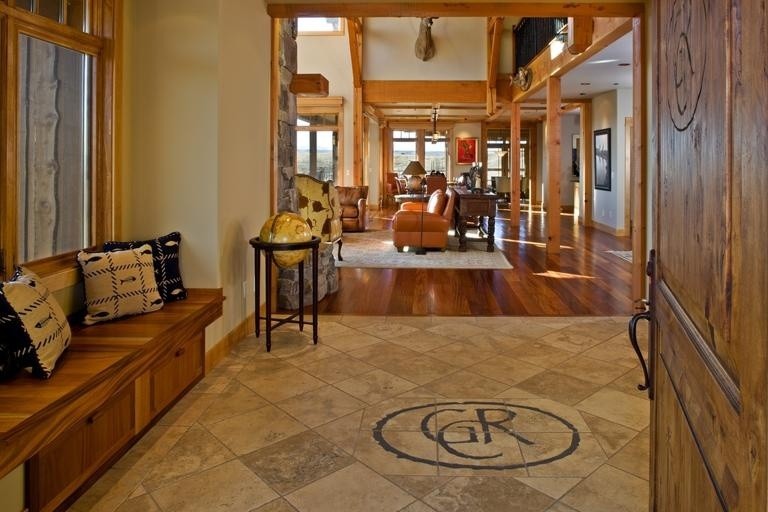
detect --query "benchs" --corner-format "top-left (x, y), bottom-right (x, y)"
top-left (0, 261), bottom-right (223, 512)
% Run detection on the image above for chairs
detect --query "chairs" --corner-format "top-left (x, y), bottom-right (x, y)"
top-left (386, 173), bottom-right (454, 254)
top-left (296, 173), bottom-right (344, 262)
top-left (332, 186), bottom-right (365, 230)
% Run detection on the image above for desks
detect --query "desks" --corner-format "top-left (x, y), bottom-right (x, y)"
top-left (451, 186), bottom-right (497, 253)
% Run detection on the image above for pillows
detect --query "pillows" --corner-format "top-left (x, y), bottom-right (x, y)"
top-left (104, 232), bottom-right (186, 303)
top-left (0, 265), bottom-right (71, 380)
top-left (78, 245), bottom-right (165, 323)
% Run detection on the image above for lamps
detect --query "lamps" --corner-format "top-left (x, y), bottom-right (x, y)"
top-left (430, 103), bottom-right (441, 143)
top-left (402, 161), bottom-right (427, 191)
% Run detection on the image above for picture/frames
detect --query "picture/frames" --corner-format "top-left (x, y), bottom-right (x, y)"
top-left (595, 129), bottom-right (611, 191)
top-left (455, 137), bottom-right (477, 165)
top-left (572, 134), bottom-right (580, 182)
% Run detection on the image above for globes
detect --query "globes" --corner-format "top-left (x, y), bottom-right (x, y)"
top-left (250, 211), bottom-right (322, 352)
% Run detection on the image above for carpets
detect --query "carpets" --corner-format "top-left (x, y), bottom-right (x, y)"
top-left (333, 228), bottom-right (513, 271)
